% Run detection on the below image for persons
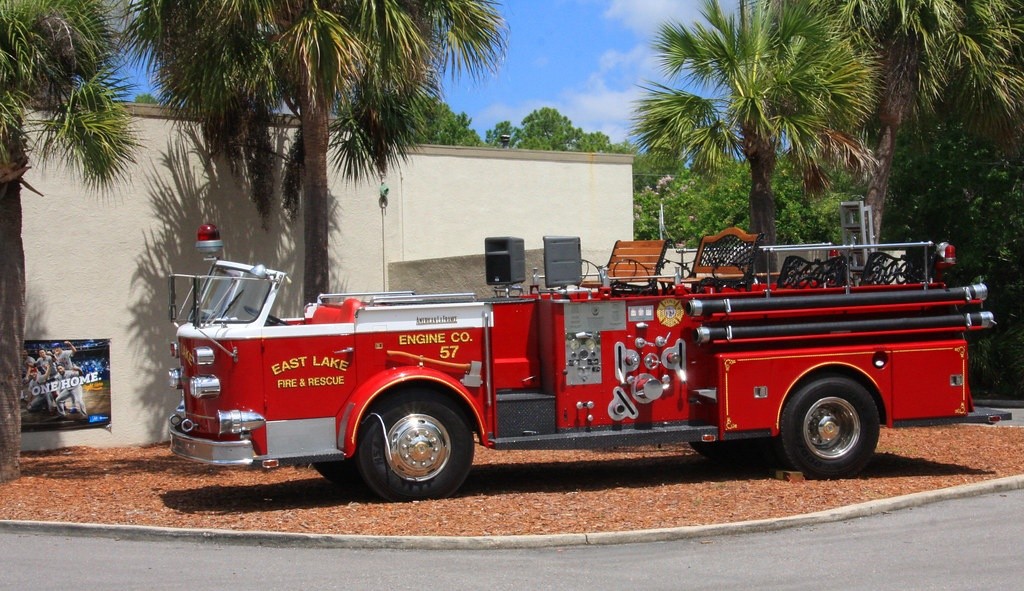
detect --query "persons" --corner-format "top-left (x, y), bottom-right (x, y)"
top-left (20, 341), bottom-right (88, 419)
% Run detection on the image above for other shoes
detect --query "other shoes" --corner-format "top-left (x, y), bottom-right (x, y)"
top-left (68, 407), bottom-right (77, 413)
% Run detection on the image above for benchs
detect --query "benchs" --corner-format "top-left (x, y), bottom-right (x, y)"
top-left (582, 239), bottom-right (669, 297)
top-left (303, 298), bottom-right (367, 325)
top-left (858, 250), bottom-right (940, 287)
top-left (776, 255), bottom-right (853, 288)
top-left (658, 228), bottom-right (766, 293)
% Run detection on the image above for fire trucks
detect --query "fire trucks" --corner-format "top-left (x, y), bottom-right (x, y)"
top-left (161, 226), bottom-right (998, 482)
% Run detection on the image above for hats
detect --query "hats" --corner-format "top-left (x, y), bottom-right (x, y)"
top-left (30, 368), bottom-right (37, 372)
top-left (51, 343), bottom-right (63, 348)
